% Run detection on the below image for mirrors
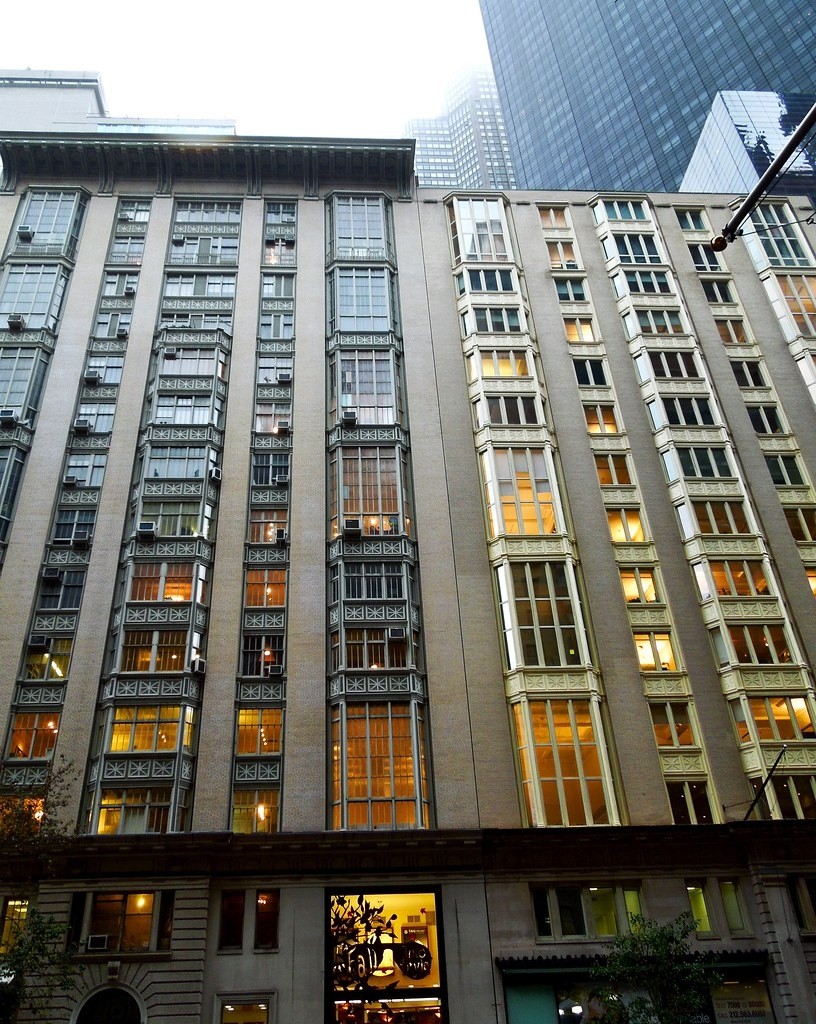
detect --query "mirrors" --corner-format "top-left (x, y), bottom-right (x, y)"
top-left (401, 926), bottom-right (428, 972)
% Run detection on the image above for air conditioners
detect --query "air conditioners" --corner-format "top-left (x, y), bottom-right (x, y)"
top-left (63, 475), bottom-right (78, 487)
top-left (276, 475), bottom-right (288, 486)
top-left (83, 328), bottom-right (291, 384)
top-left (192, 658), bottom-right (206, 673)
top-left (269, 665), bottom-right (283, 675)
top-left (116, 211), bottom-right (295, 296)
top-left (388, 627), bottom-right (407, 641)
top-left (74, 419), bottom-right (91, 432)
top-left (275, 528), bottom-right (285, 542)
top-left (17, 224), bottom-right (33, 239)
top-left (341, 411), bottom-right (357, 424)
top-left (343, 519), bottom-right (362, 535)
top-left (28, 635), bottom-right (49, 649)
top-left (209, 467), bottom-right (222, 481)
top-left (138, 522), bottom-right (157, 535)
top-left (72, 531), bottom-right (89, 542)
top-left (0, 312), bottom-right (33, 423)
top-left (278, 422), bottom-right (289, 433)
top-left (42, 567), bottom-right (62, 583)
top-left (89, 935), bottom-right (109, 951)
